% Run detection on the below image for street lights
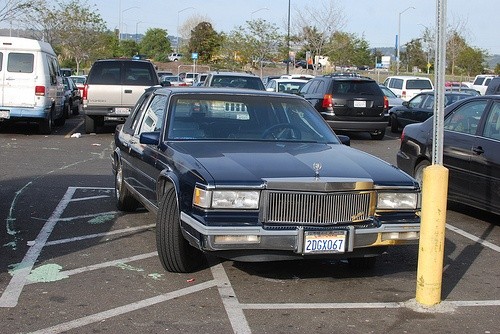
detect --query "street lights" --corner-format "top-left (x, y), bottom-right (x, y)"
top-left (121, 6), bottom-right (140, 39)
top-left (136, 22), bottom-right (143, 44)
top-left (177, 6), bottom-right (195, 54)
top-left (397, 6), bottom-right (416, 75)
top-left (251, 7), bottom-right (270, 20)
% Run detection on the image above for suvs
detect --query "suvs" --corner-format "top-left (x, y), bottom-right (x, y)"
top-left (291, 73), bottom-right (391, 140)
top-left (81, 58), bottom-right (162, 133)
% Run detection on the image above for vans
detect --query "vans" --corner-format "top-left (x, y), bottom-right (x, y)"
top-left (0, 36), bottom-right (72, 133)
top-left (384, 76), bottom-right (434, 103)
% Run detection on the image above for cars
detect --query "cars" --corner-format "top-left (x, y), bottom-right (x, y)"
top-left (293, 59), bottom-right (317, 70)
top-left (61, 67), bottom-right (88, 118)
top-left (111, 85), bottom-right (422, 276)
top-left (168, 53), bottom-right (183, 62)
top-left (358, 65), bottom-right (369, 71)
top-left (445, 74), bottom-right (500, 96)
top-left (390, 91), bottom-right (476, 133)
top-left (266, 74), bottom-right (315, 95)
top-left (158, 71), bottom-right (266, 92)
top-left (377, 83), bottom-right (404, 125)
top-left (397, 93), bottom-right (500, 215)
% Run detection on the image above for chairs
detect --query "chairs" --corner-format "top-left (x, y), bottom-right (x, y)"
top-left (169, 118), bottom-right (205, 140)
top-left (241, 121), bottom-right (275, 140)
top-left (103, 72), bottom-right (116, 82)
